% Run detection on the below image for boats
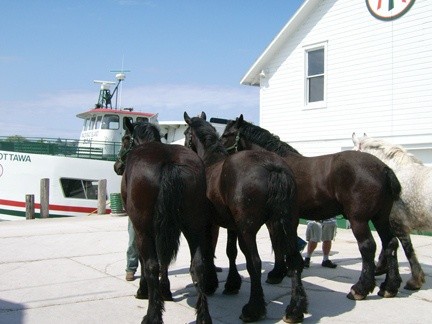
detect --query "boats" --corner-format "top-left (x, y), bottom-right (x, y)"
top-left (0, 55), bottom-right (233, 220)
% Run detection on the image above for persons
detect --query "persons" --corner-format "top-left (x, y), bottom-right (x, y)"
top-left (126, 216), bottom-right (139, 279)
top-left (304, 215), bottom-right (338, 269)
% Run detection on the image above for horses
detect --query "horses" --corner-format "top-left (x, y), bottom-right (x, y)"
top-left (177, 111), bottom-right (308, 320)
top-left (352, 130), bottom-right (432, 290)
top-left (219, 114), bottom-right (402, 301)
top-left (112, 116), bottom-right (212, 324)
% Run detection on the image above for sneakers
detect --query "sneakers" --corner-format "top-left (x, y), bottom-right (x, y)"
top-left (322, 260), bottom-right (337, 268)
top-left (126, 271), bottom-right (135, 281)
top-left (304, 257), bottom-right (310, 268)
